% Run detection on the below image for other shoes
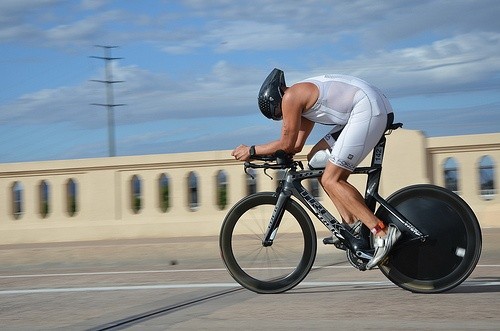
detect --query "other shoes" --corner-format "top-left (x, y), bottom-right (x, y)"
top-left (323, 220), bottom-right (363, 244)
top-left (366, 223), bottom-right (401, 270)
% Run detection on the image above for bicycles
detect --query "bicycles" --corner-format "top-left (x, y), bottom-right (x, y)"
top-left (219, 123), bottom-right (483, 296)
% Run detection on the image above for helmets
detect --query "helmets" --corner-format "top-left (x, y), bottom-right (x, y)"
top-left (258, 68), bottom-right (286, 120)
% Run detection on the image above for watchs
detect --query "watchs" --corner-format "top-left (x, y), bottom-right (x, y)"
top-left (249, 145), bottom-right (256, 158)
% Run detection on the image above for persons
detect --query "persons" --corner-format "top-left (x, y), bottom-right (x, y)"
top-left (231, 68), bottom-right (402, 272)
